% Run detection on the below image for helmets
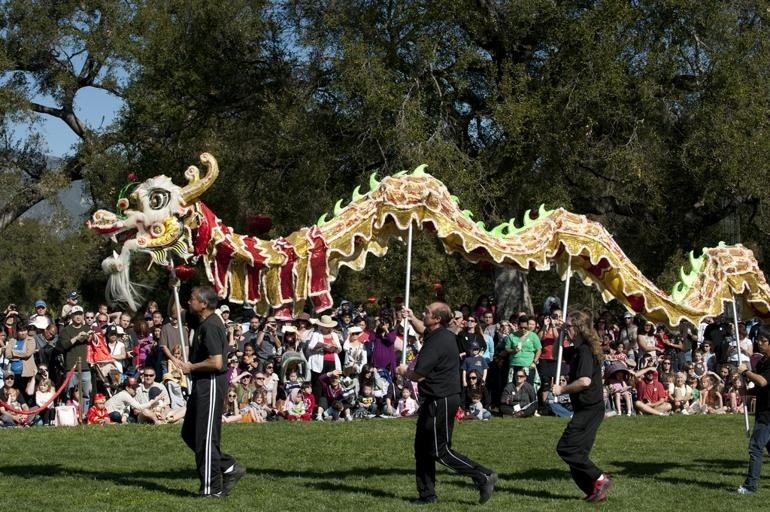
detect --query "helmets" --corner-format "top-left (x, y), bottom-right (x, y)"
top-left (467, 342), bottom-right (480, 355)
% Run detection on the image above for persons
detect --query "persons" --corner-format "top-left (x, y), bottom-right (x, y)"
top-left (168, 274), bottom-right (246, 500)
top-left (395, 302), bottom-right (498, 505)
top-left (551, 310), bottom-right (613, 502)
top-left (737, 325), bottom-right (770, 494)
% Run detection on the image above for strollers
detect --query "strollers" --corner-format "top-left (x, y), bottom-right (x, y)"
top-left (280, 352), bottom-right (313, 405)
top-left (602, 358), bottom-right (643, 417)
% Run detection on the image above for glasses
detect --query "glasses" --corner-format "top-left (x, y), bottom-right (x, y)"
top-left (144, 374), bottom-right (155, 377)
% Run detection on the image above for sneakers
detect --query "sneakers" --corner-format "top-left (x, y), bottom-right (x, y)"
top-left (582, 473), bottom-right (613, 503)
top-left (411, 495), bottom-right (438, 504)
top-left (198, 463), bottom-right (246, 498)
top-left (479, 472), bottom-right (498, 504)
top-left (736, 485), bottom-right (751, 493)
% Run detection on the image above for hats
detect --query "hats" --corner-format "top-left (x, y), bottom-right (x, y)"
top-left (221, 304), bottom-right (230, 314)
top-left (149, 387), bottom-right (163, 401)
top-left (72, 305), bottom-right (83, 313)
top-left (35, 299), bottom-right (47, 308)
top-left (67, 290), bottom-right (79, 299)
top-left (94, 393), bottom-right (106, 403)
top-left (3, 371), bottom-right (15, 380)
top-left (294, 312), bottom-right (338, 328)
top-left (123, 376), bottom-right (140, 385)
